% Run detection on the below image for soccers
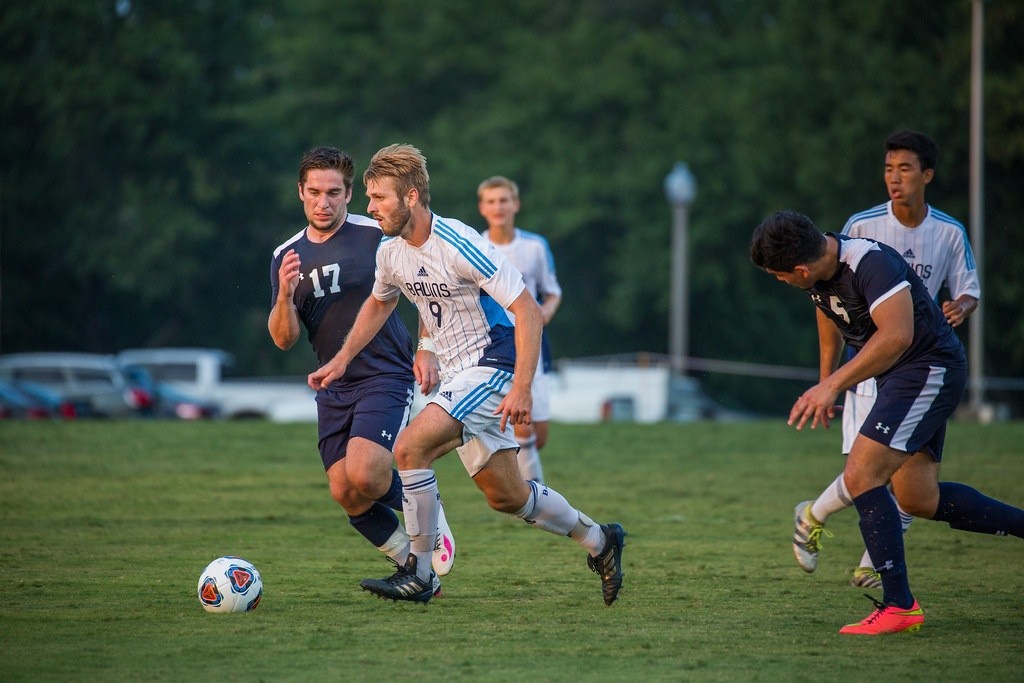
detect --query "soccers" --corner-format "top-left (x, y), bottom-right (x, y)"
top-left (197, 556), bottom-right (263, 613)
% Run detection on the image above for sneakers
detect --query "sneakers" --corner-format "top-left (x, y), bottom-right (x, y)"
top-left (839, 594), bottom-right (925, 635)
top-left (794, 500), bottom-right (834, 574)
top-left (851, 567), bottom-right (883, 588)
top-left (431, 570), bottom-right (441, 596)
top-left (432, 500), bottom-right (455, 576)
top-left (360, 552), bottom-right (434, 605)
top-left (587, 524), bottom-right (627, 607)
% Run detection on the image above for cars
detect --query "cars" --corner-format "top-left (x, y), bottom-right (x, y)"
top-left (0, 350), bottom-right (223, 421)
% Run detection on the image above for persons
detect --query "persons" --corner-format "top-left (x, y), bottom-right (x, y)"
top-left (304, 142), bottom-right (624, 610)
top-left (265, 147), bottom-right (456, 599)
top-left (467, 175), bottom-right (562, 489)
top-left (748, 209), bottom-right (1024, 637)
top-left (792, 130), bottom-right (981, 591)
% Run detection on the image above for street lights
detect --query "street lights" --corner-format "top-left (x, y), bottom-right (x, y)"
top-left (663, 160), bottom-right (697, 418)
